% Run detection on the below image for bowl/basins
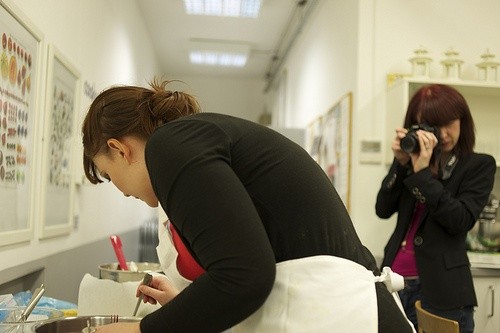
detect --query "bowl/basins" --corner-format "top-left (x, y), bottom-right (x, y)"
top-left (98, 262), bottom-right (162, 284)
top-left (0, 306), bottom-right (64, 333)
top-left (33, 315), bottom-right (144, 333)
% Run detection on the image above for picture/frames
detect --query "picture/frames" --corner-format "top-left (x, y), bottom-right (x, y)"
top-left (40, 45), bottom-right (82, 238)
top-left (305, 93), bottom-right (350, 215)
top-left (0, 1), bottom-right (45, 246)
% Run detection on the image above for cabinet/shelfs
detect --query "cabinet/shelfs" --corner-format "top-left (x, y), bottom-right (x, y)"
top-left (388, 76), bottom-right (500, 333)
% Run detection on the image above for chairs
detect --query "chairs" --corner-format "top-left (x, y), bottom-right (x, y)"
top-left (414, 300), bottom-right (459, 333)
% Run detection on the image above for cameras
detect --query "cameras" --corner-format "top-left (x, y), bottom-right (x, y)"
top-left (399, 125), bottom-right (439, 154)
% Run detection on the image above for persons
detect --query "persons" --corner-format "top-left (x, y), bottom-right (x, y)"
top-left (158, 202), bottom-right (206, 292)
top-left (81, 73), bottom-right (418, 333)
top-left (375, 84), bottom-right (498, 333)
